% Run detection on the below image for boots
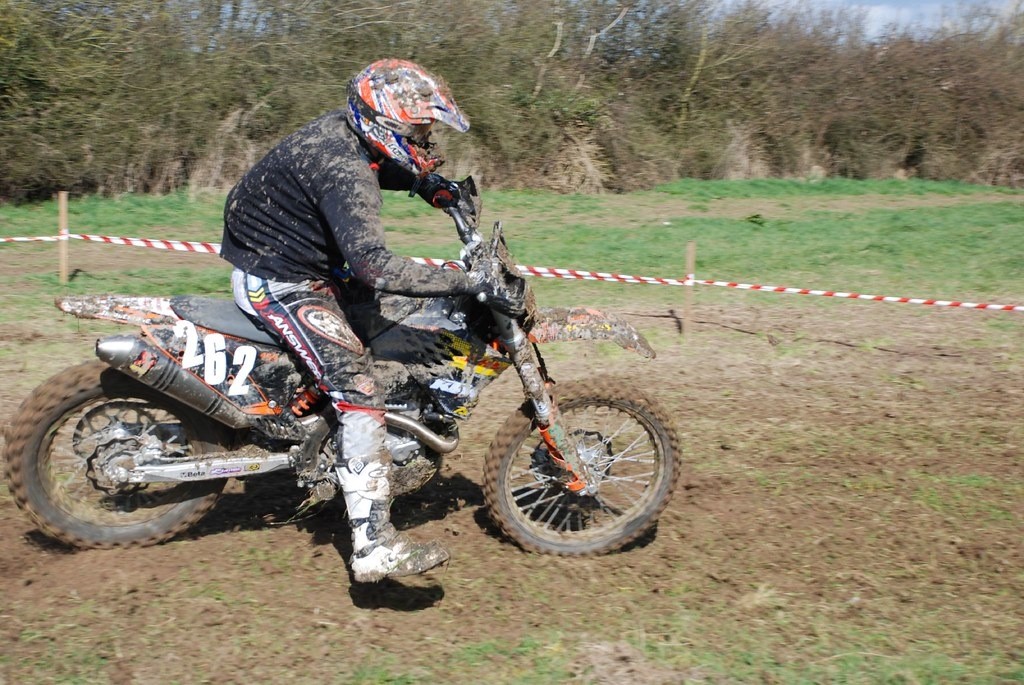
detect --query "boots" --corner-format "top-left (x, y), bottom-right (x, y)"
top-left (332, 450), bottom-right (450, 582)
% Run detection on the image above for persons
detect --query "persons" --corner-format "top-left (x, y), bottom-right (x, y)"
top-left (220, 57), bottom-right (511, 584)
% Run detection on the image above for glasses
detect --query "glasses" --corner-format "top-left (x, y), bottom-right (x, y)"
top-left (410, 124), bottom-right (433, 140)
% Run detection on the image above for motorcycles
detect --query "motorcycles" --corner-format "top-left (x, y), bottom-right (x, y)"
top-left (0, 168), bottom-right (683, 559)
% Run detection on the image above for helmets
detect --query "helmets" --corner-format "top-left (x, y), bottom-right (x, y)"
top-left (347, 59), bottom-right (470, 179)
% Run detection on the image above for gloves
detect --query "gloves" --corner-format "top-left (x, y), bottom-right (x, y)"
top-left (419, 173), bottom-right (459, 208)
top-left (462, 264), bottom-right (497, 304)
